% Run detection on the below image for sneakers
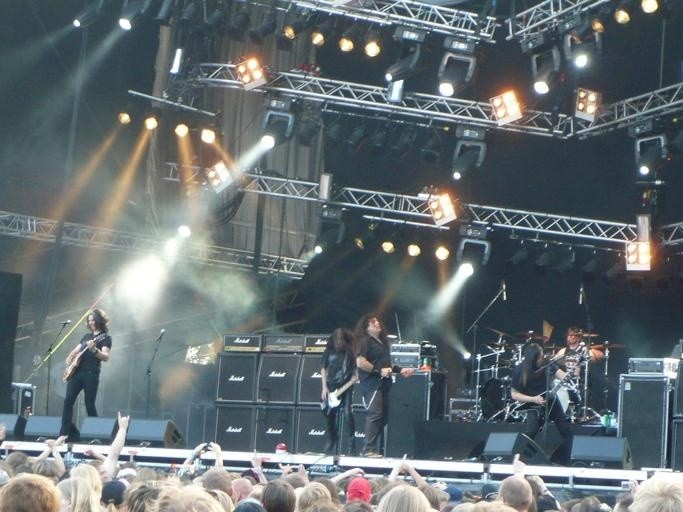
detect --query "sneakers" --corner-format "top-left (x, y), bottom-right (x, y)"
top-left (361, 450), bottom-right (384, 459)
top-left (511, 454), bottom-right (528, 467)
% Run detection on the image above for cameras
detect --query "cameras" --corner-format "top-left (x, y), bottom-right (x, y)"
top-left (202, 444), bottom-right (212, 451)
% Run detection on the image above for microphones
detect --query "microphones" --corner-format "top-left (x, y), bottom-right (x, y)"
top-left (59, 319), bottom-right (72, 325)
top-left (155, 328), bottom-right (165, 343)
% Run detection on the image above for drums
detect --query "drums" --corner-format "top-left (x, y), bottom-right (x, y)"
top-left (479, 377), bottom-right (522, 422)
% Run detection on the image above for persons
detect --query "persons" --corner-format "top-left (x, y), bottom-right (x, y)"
top-left (319, 327), bottom-right (359, 455)
top-left (355, 313), bottom-right (414, 457)
top-left (510, 343), bottom-right (573, 466)
top-left (549, 327), bottom-right (604, 422)
top-left (0, 412), bottom-right (683, 511)
top-left (59, 308), bottom-right (112, 437)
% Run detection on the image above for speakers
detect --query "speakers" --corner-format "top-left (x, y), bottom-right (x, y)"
top-left (483, 430), bottom-right (545, 465)
top-left (671, 418), bottom-right (683, 472)
top-left (24, 415), bottom-right (80, 443)
top-left (385, 369), bottom-right (446, 459)
top-left (79, 415), bottom-right (118, 443)
top-left (0, 413), bottom-right (27, 440)
top-left (570, 434), bottom-right (634, 471)
top-left (673, 360), bottom-right (683, 417)
top-left (616, 372), bottom-right (673, 471)
top-left (126, 418), bottom-right (186, 448)
top-left (214, 333), bottom-right (384, 458)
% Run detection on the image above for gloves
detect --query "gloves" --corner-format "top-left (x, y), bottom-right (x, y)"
top-left (65, 452), bottom-right (75, 468)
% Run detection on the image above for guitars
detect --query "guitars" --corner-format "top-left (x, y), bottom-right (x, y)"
top-left (63, 333), bottom-right (106, 382)
top-left (321, 377), bottom-right (357, 416)
top-left (514, 351), bottom-right (592, 431)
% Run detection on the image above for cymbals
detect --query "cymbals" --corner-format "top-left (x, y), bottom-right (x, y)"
top-left (583, 333), bottom-right (599, 338)
top-left (589, 343), bottom-right (626, 349)
top-left (514, 332), bottom-right (548, 340)
top-left (489, 329), bottom-right (517, 339)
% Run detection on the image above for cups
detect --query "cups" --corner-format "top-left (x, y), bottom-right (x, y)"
top-left (605, 410), bottom-right (617, 429)
top-left (423, 358), bottom-right (433, 370)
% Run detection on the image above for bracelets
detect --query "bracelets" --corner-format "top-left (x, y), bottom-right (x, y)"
top-left (94, 348), bottom-right (99, 356)
top-left (370, 366), bottom-right (381, 377)
top-left (392, 365), bottom-right (401, 373)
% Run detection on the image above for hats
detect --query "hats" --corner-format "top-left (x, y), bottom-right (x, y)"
top-left (537, 494), bottom-right (558, 512)
top-left (442, 486), bottom-right (463, 505)
top-left (346, 478), bottom-right (372, 501)
top-left (101, 478), bottom-right (130, 505)
top-left (481, 482), bottom-right (498, 499)
top-left (241, 468), bottom-right (260, 484)
top-left (116, 468), bottom-right (136, 479)
